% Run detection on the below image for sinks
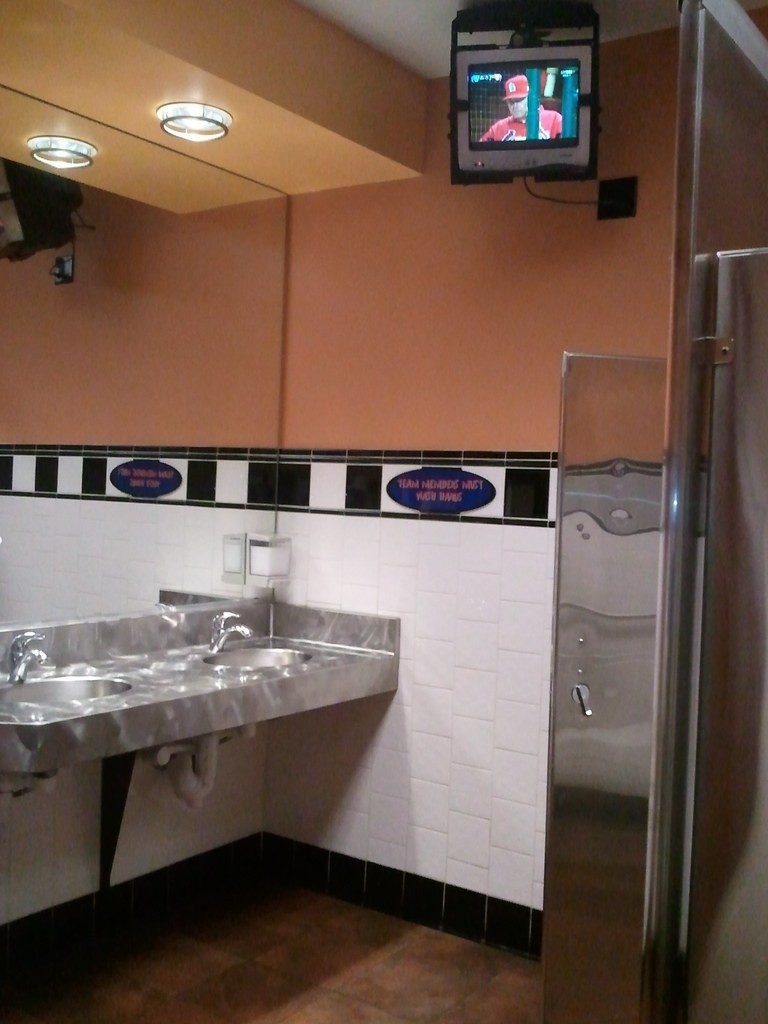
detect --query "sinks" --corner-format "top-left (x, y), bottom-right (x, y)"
top-left (201, 643), bottom-right (315, 671)
top-left (0, 676), bottom-right (135, 707)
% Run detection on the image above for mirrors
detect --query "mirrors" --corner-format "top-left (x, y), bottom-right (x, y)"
top-left (0, 86), bottom-right (289, 629)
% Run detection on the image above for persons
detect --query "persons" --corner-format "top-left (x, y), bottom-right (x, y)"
top-left (478, 75), bottom-right (562, 142)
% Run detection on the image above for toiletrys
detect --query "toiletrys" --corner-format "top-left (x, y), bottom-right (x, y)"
top-left (220, 533), bottom-right (246, 586)
top-left (246, 533), bottom-right (292, 589)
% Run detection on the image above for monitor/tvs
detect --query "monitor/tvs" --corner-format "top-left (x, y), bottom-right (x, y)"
top-left (455, 44), bottom-right (591, 172)
top-left (0, 156), bottom-right (84, 262)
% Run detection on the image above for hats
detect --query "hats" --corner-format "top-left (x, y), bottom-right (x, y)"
top-left (501, 75), bottom-right (529, 101)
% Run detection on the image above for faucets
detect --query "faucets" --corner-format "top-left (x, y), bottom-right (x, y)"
top-left (9, 630), bottom-right (48, 686)
top-left (208, 609), bottom-right (249, 651)
